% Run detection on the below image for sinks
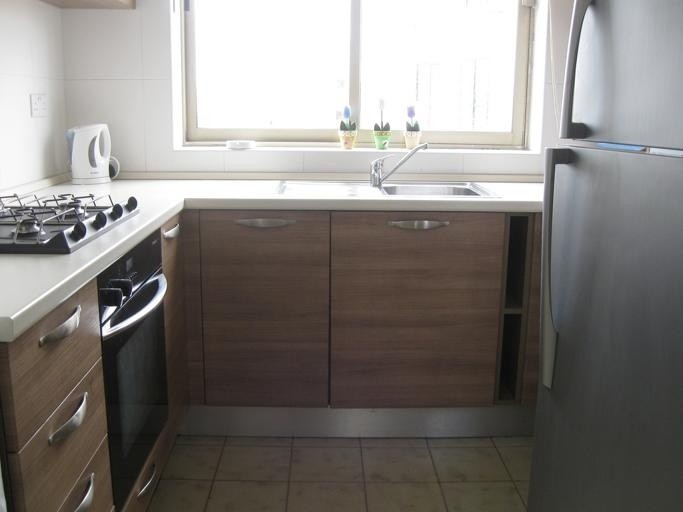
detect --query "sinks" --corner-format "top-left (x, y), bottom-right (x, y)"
top-left (376, 181), bottom-right (494, 197)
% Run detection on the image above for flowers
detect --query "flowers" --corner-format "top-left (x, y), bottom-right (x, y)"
top-left (340, 105), bottom-right (356, 131)
top-left (374, 99), bottom-right (390, 131)
top-left (405, 107), bottom-right (421, 131)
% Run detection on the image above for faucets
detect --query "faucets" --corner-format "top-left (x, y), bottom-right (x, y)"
top-left (369, 143), bottom-right (428, 187)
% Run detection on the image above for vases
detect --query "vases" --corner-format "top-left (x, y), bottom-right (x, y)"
top-left (339, 131), bottom-right (356, 149)
top-left (403, 131), bottom-right (421, 149)
top-left (373, 131), bottom-right (391, 149)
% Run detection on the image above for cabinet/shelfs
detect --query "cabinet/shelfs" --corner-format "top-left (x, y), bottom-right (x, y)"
top-left (330, 211), bottom-right (505, 407)
top-left (42, 0), bottom-right (137, 10)
top-left (122, 425), bottom-right (176, 512)
top-left (162, 210), bottom-right (184, 419)
top-left (183, 208), bottom-right (329, 409)
top-left (1, 277), bottom-right (116, 512)
top-left (500, 214), bottom-right (542, 406)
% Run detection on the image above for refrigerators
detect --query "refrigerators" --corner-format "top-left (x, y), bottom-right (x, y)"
top-left (525, 0), bottom-right (683, 512)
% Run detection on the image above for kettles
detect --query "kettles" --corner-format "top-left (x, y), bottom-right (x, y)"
top-left (67, 123), bottom-right (112, 185)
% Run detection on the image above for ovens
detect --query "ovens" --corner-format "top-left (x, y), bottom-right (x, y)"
top-left (95, 229), bottom-right (171, 511)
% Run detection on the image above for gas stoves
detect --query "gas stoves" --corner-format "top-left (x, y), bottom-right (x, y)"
top-left (0, 192), bottom-right (140, 253)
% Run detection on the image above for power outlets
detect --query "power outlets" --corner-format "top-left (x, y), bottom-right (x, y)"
top-left (30, 92), bottom-right (49, 116)
